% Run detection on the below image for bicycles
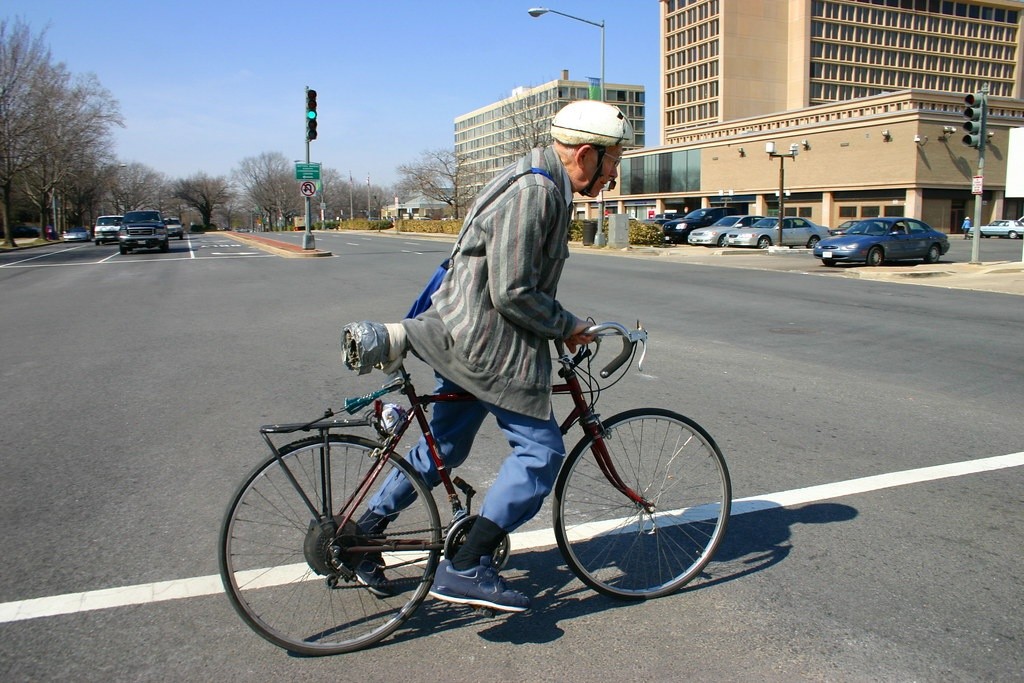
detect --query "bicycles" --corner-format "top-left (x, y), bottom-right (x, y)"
top-left (218, 321), bottom-right (732, 659)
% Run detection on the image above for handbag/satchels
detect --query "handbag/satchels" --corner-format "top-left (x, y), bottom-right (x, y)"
top-left (403, 257), bottom-right (453, 319)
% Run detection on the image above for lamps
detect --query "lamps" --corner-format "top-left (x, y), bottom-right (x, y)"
top-left (881, 129), bottom-right (890, 143)
top-left (801, 139), bottom-right (812, 151)
top-left (938, 125), bottom-right (957, 141)
top-left (984, 132), bottom-right (996, 143)
top-left (737, 145), bottom-right (745, 159)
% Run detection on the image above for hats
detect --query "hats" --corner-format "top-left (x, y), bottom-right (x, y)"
top-left (965, 217), bottom-right (970, 219)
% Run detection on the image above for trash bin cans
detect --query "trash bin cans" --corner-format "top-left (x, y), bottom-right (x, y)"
top-left (582, 220), bottom-right (597, 246)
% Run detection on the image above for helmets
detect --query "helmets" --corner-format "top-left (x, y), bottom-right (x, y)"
top-left (550, 100), bottom-right (636, 148)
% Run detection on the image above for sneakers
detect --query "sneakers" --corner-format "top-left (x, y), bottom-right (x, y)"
top-left (428, 555), bottom-right (530, 612)
top-left (354, 555), bottom-right (396, 596)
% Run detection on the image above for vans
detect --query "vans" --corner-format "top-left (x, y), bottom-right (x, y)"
top-left (92, 215), bottom-right (124, 245)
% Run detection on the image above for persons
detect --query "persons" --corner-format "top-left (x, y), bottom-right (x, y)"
top-left (355, 99), bottom-right (632, 611)
top-left (961, 217), bottom-right (971, 239)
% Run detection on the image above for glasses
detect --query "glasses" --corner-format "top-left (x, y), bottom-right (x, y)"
top-left (595, 151), bottom-right (622, 167)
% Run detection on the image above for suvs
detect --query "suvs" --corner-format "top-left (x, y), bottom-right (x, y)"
top-left (164, 217), bottom-right (185, 240)
top-left (114, 209), bottom-right (172, 255)
top-left (662, 206), bottom-right (743, 243)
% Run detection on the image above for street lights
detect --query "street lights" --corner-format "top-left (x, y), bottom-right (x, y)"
top-left (765, 141), bottom-right (798, 248)
top-left (527, 6), bottom-right (606, 248)
top-left (293, 158), bottom-right (326, 231)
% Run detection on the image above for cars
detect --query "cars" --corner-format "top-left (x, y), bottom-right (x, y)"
top-left (8, 225), bottom-right (41, 238)
top-left (723, 216), bottom-right (831, 249)
top-left (654, 212), bottom-right (686, 225)
top-left (830, 220), bottom-right (882, 237)
top-left (687, 215), bottom-right (766, 248)
top-left (813, 216), bottom-right (951, 267)
top-left (999, 216), bottom-right (1024, 226)
top-left (967, 220), bottom-right (1024, 240)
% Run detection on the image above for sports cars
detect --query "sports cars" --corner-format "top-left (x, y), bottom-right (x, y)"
top-left (62, 227), bottom-right (91, 242)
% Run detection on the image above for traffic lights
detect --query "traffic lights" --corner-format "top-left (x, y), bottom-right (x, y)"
top-left (961, 92), bottom-right (985, 149)
top-left (606, 180), bottom-right (617, 190)
top-left (305, 89), bottom-right (318, 141)
